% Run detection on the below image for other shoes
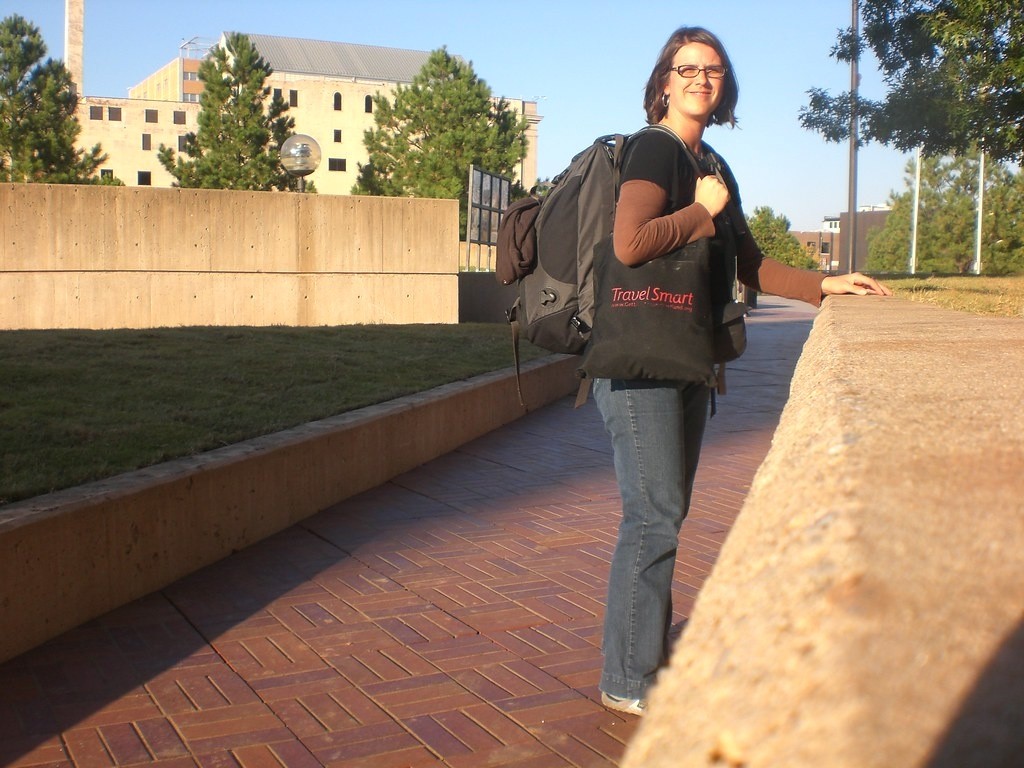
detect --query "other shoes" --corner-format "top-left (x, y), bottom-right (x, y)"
top-left (602, 692), bottom-right (648, 716)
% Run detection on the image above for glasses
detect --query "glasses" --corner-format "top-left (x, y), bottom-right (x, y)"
top-left (671, 64), bottom-right (728, 78)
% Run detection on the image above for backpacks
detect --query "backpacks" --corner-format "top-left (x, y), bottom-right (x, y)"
top-left (496, 124), bottom-right (734, 355)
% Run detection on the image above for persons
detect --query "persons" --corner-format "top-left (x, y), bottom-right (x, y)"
top-left (577, 27), bottom-right (891, 718)
